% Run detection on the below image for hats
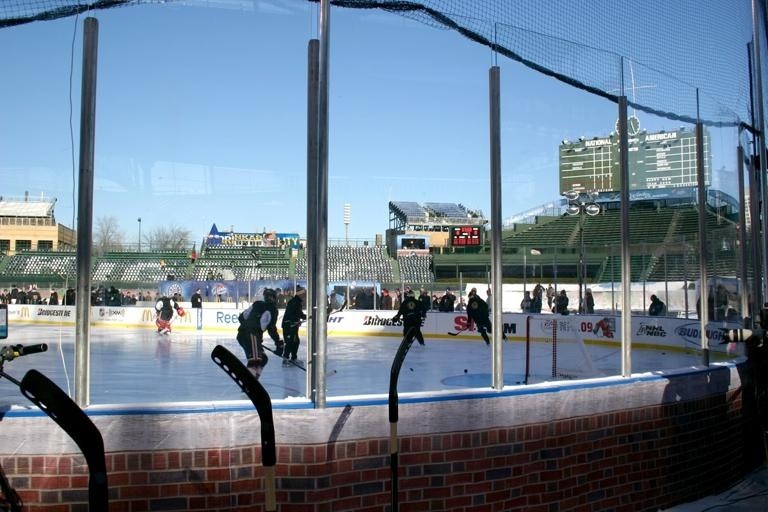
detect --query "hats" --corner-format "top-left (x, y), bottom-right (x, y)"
top-left (408, 290), bottom-right (415, 298)
top-left (296, 285), bottom-right (305, 295)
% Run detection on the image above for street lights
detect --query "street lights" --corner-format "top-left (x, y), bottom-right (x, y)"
top-left (138, 217), bottom-right (141, 252)
top-left (344, 202), bottom-right (351, 246)
top-left (564, 188), bottom-right (601, 313)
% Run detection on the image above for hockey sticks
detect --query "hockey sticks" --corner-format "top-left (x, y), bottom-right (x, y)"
top-left (448, 327), bottom-right (468, 335)
top-left (212, 346), bottom-right (277, 510)
top-left (389, 327), bottom-right (417, 512)
top-left (21, 369), bottom-right (109, 512)
top-left (261, 344), bottom-right (337, 379)
top-left (326, 405), bottom-right (356, 473)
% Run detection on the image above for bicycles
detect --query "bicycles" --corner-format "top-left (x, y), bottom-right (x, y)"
top-left (0, 342), bottom-right (48, 510)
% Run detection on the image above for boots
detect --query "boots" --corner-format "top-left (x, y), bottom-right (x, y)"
top-left (283, 357), bottom-right (303, 365)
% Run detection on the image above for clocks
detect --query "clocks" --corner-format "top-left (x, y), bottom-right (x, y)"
top-left (627, 115), bottom-right (640, 137)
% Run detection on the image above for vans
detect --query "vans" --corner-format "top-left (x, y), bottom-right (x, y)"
top-left (694, 275), bottom-right (767, 323)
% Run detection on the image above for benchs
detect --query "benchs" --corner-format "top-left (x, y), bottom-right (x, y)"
top-left (488, 204), bottom-right (764, 281)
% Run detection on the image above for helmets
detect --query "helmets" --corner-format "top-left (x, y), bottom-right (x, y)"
top-left (263, 289), bottom-right (277, 305)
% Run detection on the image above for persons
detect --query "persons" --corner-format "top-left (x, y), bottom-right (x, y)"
top-left (155, 293), bottom-right (184, 335)
top-left (1, 285), bottom-right (158, 307)
top-left (191, 288), bottom-right (202, 308)
top-left (649, 284), bottom-right (727, 321)
top-left (237, 285), bottom-right (305, 380)
top-left (519, 282), bottom-right (594, 316)
top-left (327, 284), bottom-right (508, 348)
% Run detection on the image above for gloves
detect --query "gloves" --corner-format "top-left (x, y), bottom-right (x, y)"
top-left (274, 340), bottom-right (284, 355)
top-left (178, 308), bottom-right (184, 316)
